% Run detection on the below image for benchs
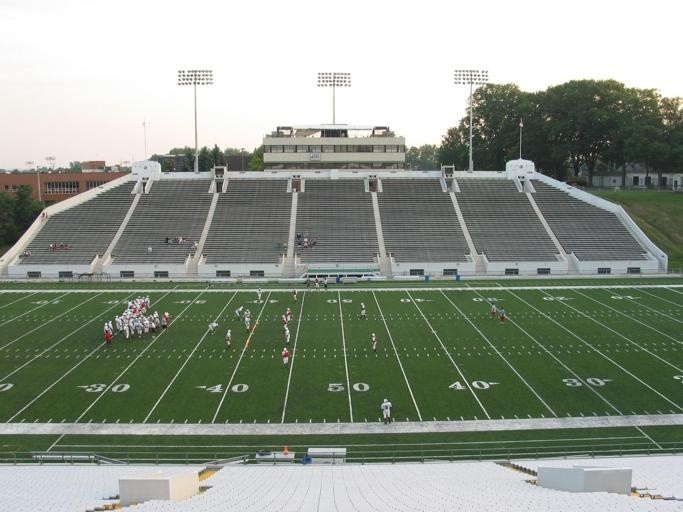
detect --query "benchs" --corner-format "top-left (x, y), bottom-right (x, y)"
top-left (0, 452), bottom-right (682, 512)
top-left (8, 169), bottom-right (657, 272)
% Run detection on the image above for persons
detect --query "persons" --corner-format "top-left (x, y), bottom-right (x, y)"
top-left (371, 333), bottom-right (377, 352)
top-left (360, 302), bottom-right (366, 319)
top-left (22, 241), bottom-right (72, 257)
top-left (281, 347), bottom-right (290, 368)
top-left (381, 398), bottom-right (391, 424)
top-left (499, 306), bottom-right (505, 325)
top-left (207, 275), bottom-right (329, 354)
top-left (490, 302), bottom-right (496, 318)
top-left (101, 294), bottom-right (172, 347)
top-left (295, 231), bottom-right (318, 249)
top-left (164, 232), bottom-right (198, 250)
